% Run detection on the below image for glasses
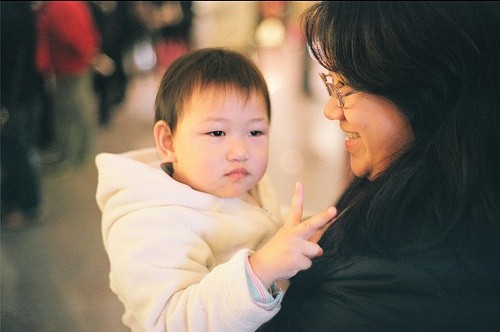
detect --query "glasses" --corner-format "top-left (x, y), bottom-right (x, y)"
top-left (320, 71), bottom-right (371, 108)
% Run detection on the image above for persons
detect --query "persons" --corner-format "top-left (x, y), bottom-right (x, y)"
top-left (254, 0), bottom-right (499, 332)
top-left (0, 0), bottom-right (201, 236)
top-left (94, 47), bottom-right (336, 332)
